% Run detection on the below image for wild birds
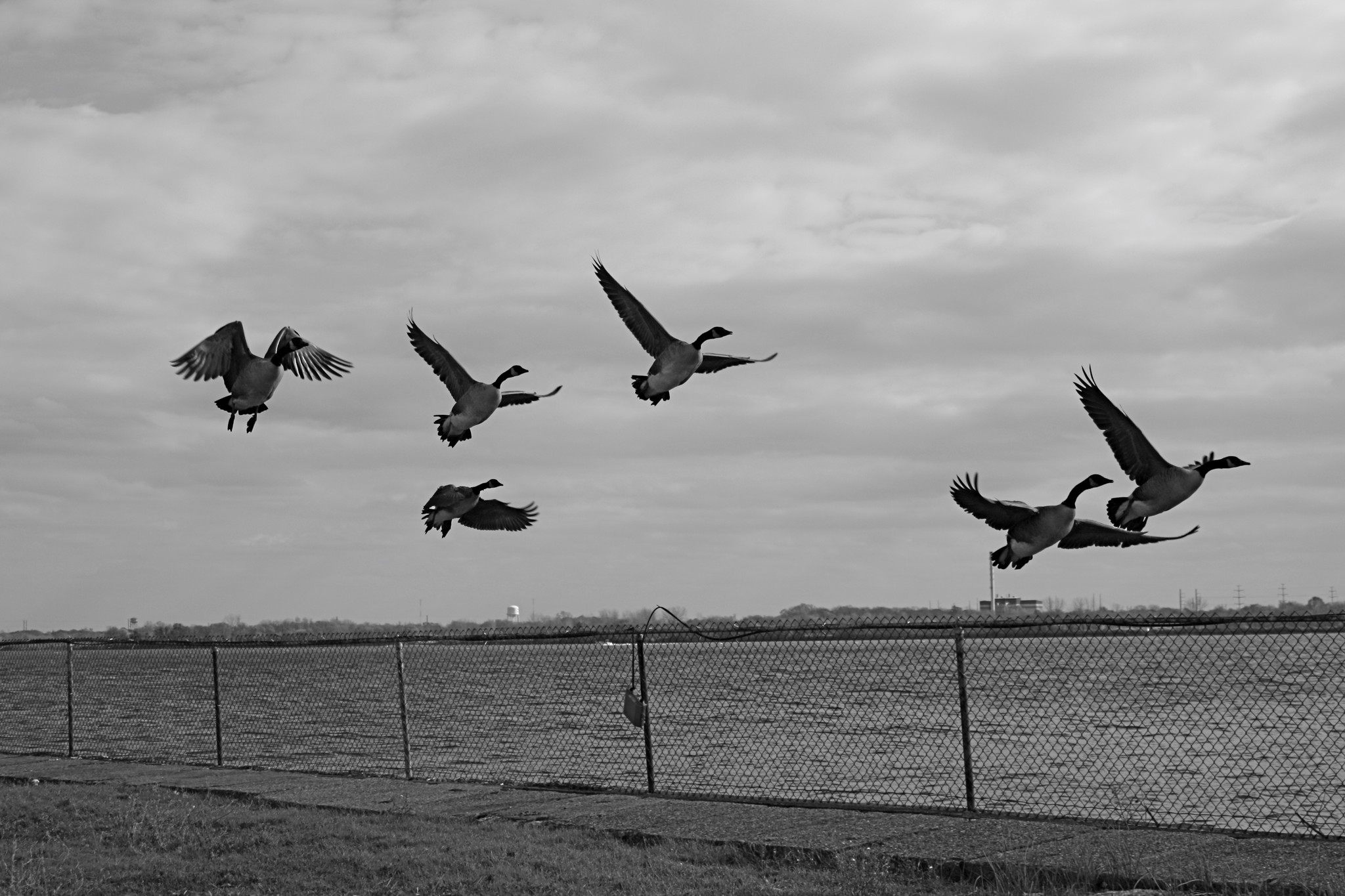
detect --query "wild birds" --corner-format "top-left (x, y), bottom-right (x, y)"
top-left (590, 250), bottom-right (778, 406)
top-left (169, 319), bottom-right (353, 434)
top-left (405, 307), bottom-right (562, 449)
top-left (420, 478), bottom-right (539, 539)
top-left (949, 471), bottom-right (1201, 571)
top-left (1073, 363), bottom-right (1251, 533)
